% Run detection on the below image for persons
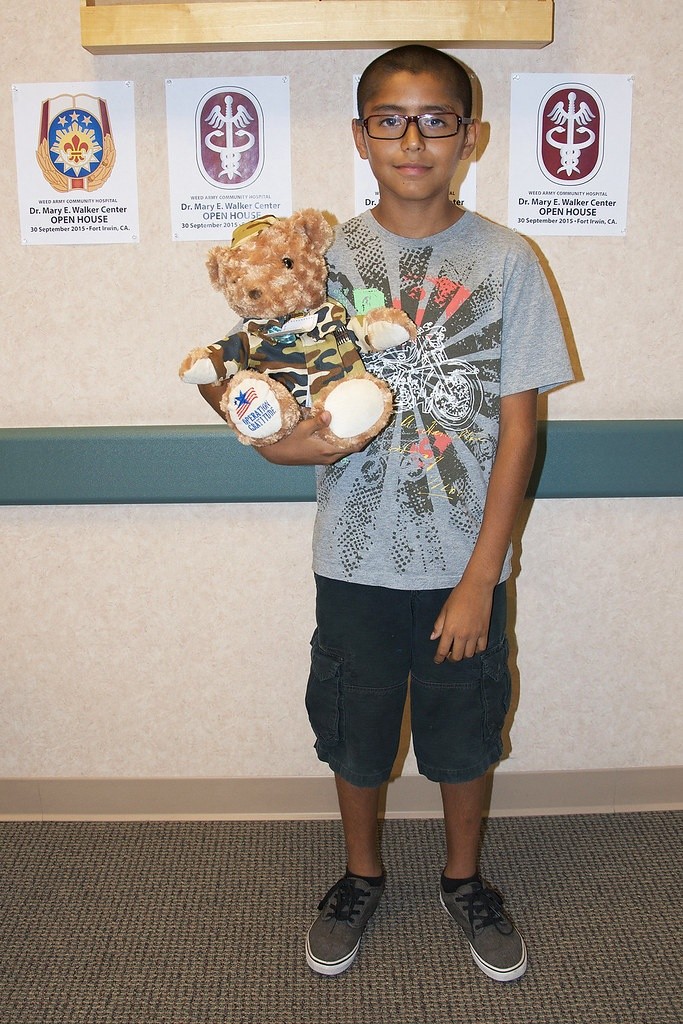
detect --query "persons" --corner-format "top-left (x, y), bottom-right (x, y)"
top-left (193, 45), bottom-right (575, 983)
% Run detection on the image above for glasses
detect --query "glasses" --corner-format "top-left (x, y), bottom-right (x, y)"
top-left (353, 111), bottom-right (476, 140)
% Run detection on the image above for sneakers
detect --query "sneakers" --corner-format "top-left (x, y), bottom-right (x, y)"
top-left (304, 867), bottom-right (387, 975)
top-left (439, 866), bottom-right (528, 982)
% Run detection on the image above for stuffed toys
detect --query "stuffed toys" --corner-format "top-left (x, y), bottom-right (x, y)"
top-left (179, 208), bottom-right (416, 453)
